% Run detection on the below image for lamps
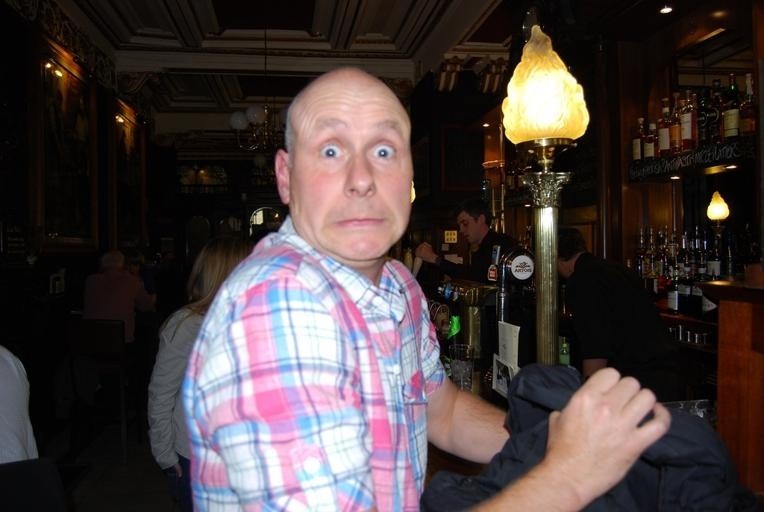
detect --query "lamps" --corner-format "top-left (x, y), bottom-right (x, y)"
top-left (706, 190), bottom-right (731, 238)
top-left (498, 21), bottom-right (590, 365)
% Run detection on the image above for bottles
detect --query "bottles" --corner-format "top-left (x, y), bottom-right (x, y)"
top-left (630, 71), bottom-right (758, 163)
top-left (437, 281), bottom-right (464, 300)
top-left (556, 291), bottom-right (581, 374)
top-left (494, 261), bottom-right (509, 324)
top-left (634, 218), bottom-right (757, 317)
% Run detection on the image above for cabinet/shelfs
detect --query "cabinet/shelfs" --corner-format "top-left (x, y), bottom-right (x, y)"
top-left (622, 0), bottom-right (764, 332)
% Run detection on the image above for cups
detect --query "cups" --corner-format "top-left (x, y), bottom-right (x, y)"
top-left (447, 344), bottom-right (472, 390)
top-left (665, 323), bottom-right (708, 347)
